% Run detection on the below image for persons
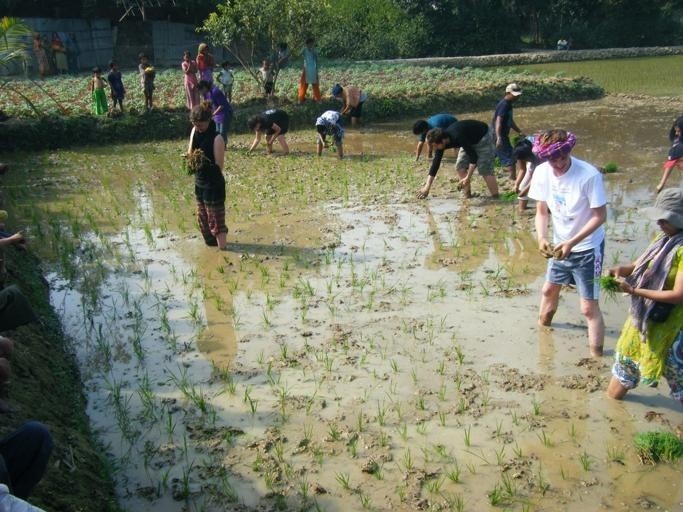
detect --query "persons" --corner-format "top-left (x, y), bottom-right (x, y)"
top-left (256, 58), bottom-right (275, 96)
top-left (34, 31), bottom-right (80, 76)
top-left (558, 37), bottom-right (567, 48)
top-left (91, 59), bottom-right (126, 115)
top-left (278, 38), bottom-right (323, 105)
top-left (652, 116), bottom-right (682, 191)
top-left (605, 188), bottom-right (683, 401)
top-left (315, 111), bottom-right (344, 158)
top-left (196, 79), bottom-right (233, 150)
top-left (331, 83), bottom-right (366, 127)
top-left (246, 109), bottom-right (290, 156)
top-left (528, 130), bottom-right (607, 356)
top-left (0, 105), bottom-right (52, 512)
top-left (181, 99), bottom-right (229, 249)
top-left (182, 44), bottom-right (234, 109)
top-left (411, 85), bottom-right (552, 210)
top-left (139, 52), bottom-right (155, 111)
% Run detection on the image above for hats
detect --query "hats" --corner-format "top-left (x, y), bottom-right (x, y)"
top-left (505, 82), bottom-right (522, 97)
top-left (638, 187), bottom-right (683, 229)
